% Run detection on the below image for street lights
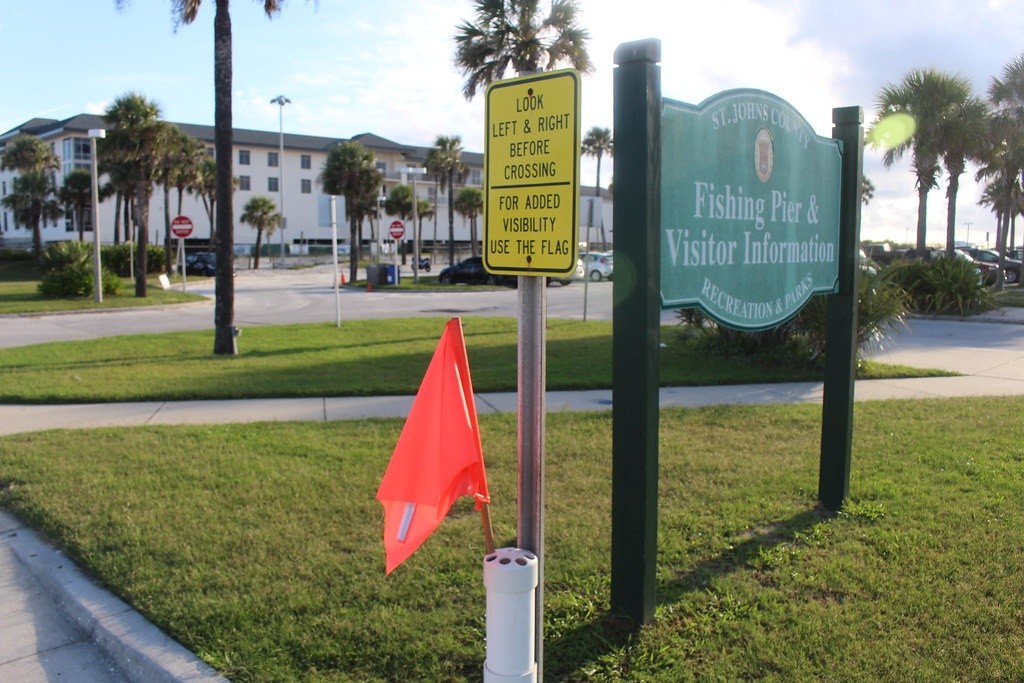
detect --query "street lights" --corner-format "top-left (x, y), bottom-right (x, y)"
top-left (87, 127), bottom-right (107, 304)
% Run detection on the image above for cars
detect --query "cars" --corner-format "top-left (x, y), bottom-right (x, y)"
top-left (546, 250), bottom-right (585, 288)
top-left (176, 252), bottom-right (236, 277)
top-left (972, 256), bottom-right (1008, 287)
top-left (902, 247), bottom-right (982, 288)
top-left (578, 251), bottom-right (614, 283)
top-left (859, 247), bottom-right (881, 277)
top-left (1013, 248), bottom-right (1024, 260)
top-left (439, 256), bottom-right (519, 289)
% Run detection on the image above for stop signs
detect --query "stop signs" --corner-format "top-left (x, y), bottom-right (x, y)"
top-left (389, 220), bottom-right (405, 239)
top-left (172, 216), bottom-right (193, 238)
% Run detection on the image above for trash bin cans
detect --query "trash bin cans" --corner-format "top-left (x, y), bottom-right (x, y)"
top-left (366, 264), bottom-right (399, 286)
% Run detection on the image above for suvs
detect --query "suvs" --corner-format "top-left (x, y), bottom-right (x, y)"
top-left (940, 244), bottom-right (1023, 284)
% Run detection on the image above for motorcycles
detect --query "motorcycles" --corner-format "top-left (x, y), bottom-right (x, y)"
top-left (411, 254), bottom-right (432, 272)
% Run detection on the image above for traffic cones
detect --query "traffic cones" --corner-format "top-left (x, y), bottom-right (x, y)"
top-left (366, 279), bottom-right (374, 292)
top-left (340, 266), bottom-right (347, 285)
